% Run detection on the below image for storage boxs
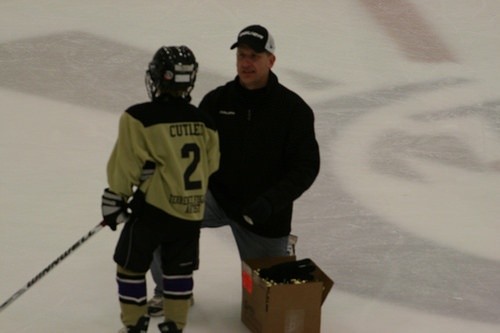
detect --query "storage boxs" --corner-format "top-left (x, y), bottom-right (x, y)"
top-left (241, 255), bottom-right (335, 333)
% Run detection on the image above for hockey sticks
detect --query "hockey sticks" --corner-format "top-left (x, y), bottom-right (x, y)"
top-left (0, 203), bottom-right (131, 310)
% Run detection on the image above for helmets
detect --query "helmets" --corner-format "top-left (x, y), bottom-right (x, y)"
top-left (148, 46), bottom-right (198, 91)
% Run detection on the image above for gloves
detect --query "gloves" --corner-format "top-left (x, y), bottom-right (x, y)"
top-left (101, 188), bottom-right (130, 230)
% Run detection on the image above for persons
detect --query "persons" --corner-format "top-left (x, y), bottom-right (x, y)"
top-left (101, 45), bottom-right (221, 333)
top-left (147, 25), bottom-right (320, 318)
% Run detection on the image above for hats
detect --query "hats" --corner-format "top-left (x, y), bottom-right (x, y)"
top-left (231, 25), bottom-right (275, 54)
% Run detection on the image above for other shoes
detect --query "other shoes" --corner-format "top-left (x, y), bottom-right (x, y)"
top-left (158, 322), bottom-right (182, 333)
top-left (119, 316), bottom-right (150, 333)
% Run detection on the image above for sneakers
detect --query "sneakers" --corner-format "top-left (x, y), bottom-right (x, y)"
top-left (148, 294), bottom-right (194, 316)
